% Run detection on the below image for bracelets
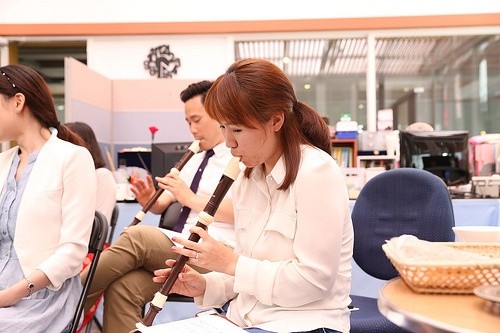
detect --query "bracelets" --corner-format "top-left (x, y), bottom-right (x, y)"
top-left (26, 279), bottom-right (34, 297)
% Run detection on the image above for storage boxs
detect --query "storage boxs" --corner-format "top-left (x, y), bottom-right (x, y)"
top-left (336, 121), bottom-right (357, 138)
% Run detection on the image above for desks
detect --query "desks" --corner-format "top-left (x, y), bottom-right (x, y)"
top-left (376, 277), bottom-right (500, 333)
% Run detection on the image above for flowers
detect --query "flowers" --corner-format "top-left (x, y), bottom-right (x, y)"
top-left (149, 126), bottom-right (158, 143)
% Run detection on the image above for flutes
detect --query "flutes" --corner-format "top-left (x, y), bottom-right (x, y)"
top-left (135, 156), bottom-right (241, 332)
top-left (128, 140), bottom-right (201, 227)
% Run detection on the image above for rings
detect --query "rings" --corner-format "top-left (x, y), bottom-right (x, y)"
top-left (196, 253), bottom-right (198, 259)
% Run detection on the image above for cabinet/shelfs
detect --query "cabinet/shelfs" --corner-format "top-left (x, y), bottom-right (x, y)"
top-left (330, 138), bottom-right (396, 170)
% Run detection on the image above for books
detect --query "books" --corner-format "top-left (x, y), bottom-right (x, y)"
top-left (329, 147), bottom-right (352, 169)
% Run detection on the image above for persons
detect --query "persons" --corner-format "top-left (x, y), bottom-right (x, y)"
top-left (64, 122), bottom-right (117, 227)
top-left (0, 65), bottom-right (97, 333)
top-left (80, 80), bottom-right (234, 333)
top-left (152, 58), bottom-right (354, 333)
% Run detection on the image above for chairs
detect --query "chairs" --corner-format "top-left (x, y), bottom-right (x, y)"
top-left (325, 167), bottom-right (453, 333)
top-left (69, 210), bottom-right (108, 333)
top-left (85, 205), bottom-right (119, 333)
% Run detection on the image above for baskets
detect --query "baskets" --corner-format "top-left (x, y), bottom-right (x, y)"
top-left (381, 240), bottom-right (500, 294)
